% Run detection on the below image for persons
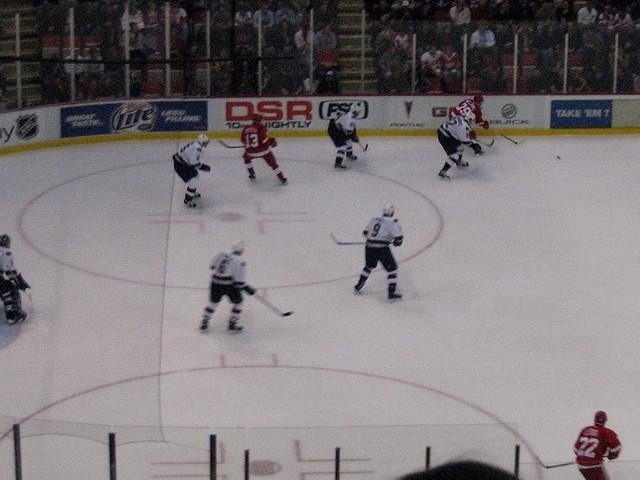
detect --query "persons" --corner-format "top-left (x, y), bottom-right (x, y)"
top-left (172, 133), bottom-right (210, 207)
top-left (197, 239), bottom-right (254, 334)
top-left (328, 102), bottom-right (363, 169)
top-left (0, 234), bottom-right (32, 327)
top-left (437, 111), bottom-right (478, 182)
top-left (449, 94), bottom-right (490, 153)
top-left (241, 111), bottom-right (288, 186)
top-left (367, 1), bottom-right (639, 94)
top-left (353, 203), bottom-right (403, 304)
top-left (572, 410), bottom-right (622, 480)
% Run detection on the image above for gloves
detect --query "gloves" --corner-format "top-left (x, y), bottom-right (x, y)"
top-left (393, 239), bottom-right (402, 247)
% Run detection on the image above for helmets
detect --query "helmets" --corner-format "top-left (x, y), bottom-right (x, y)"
top-left (383, 202), bottom-right (395, 216)
top-left (197, 134), bottom-right (209, 146)
top-left (595, 410), bottom-right (607, 422)
top-left (0, 234), bottom-right (10, 246)
top-left (351, 103), bottom-right (361, 114)
top-left (474, 95), bottom-right (483, 104)
top-left (252, 113), bottom-right (264, 122)
top-left (464, 111), bottom-right (475, 122)
top-left (231, 239), bottom-right (246, 251)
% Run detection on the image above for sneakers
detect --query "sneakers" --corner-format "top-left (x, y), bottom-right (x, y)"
top-left (388, 294), bottom-right (403, 303)
top-left (353, 286), bottom-right (364, 294)
top-left (248, 174), bottom-right (257, 181)
top-left (184, 192), bottom-right (201, 209)
top-left (456, 161), bottom-right (469, 168)
top-left (6, 310), bottom-right (29, 329)
top-left (199, 324), bottom-right (209, 332)
top-left (280, 178), bottom-right (288, 185)
top-left (346, 155), bottom-right (357, 162)
top-left (474, 151), bottom-right (485, 157)
top-left (229, 324), bottom-right (246, 333)
top-left (438, 169), bottom-right (451, 181)
top-left (335, 165), bottom-right (347, 171)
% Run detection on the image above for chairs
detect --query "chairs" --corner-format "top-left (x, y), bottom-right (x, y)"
top-left (27, 0), bottom-right (640, 105)
